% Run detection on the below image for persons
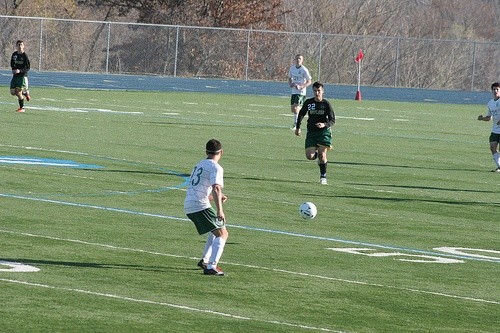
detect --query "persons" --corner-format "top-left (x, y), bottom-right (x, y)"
top-left (477, 82), bottom-right (500, 173)
top-left (10, 40), bottom-right (30, 112)
top-left (183, 139), bottom-right (229, 277)
top-left (294, 82), bottom-right (335, 185)
top-left (287, 53), bottom-right (312, 130)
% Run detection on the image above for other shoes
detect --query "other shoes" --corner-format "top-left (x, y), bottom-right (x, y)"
top-left (292, 126), bottom-right (296, 130)
top-left (495, 167), bottom-right (500, 172)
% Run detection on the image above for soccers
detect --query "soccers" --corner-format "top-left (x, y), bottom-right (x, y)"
top-left (299, 201), bottom-right (317, 219)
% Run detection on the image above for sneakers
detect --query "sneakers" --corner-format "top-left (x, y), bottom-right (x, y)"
top-left (320, 177), bottom-right (327, 184)
top-left (197, 259), bottom-right (208, 269)
top-left (25, 90), bottom-right (30, 101)
top-left (204, 268), bottom-right (224, 276)
top-left (16, 108), bottom-right (25, 112)
